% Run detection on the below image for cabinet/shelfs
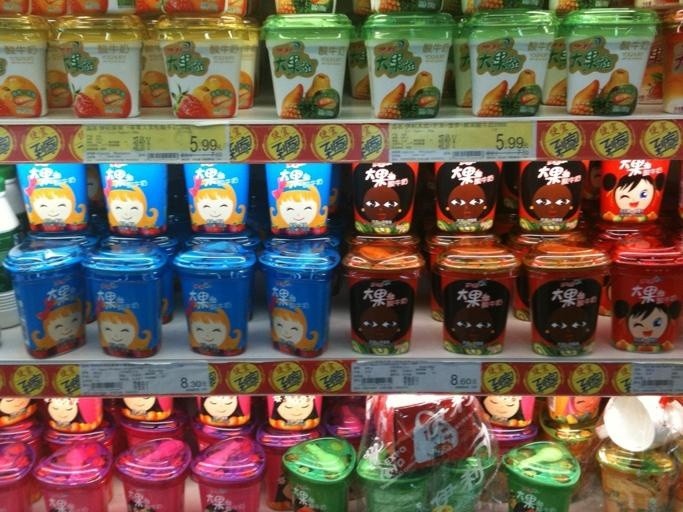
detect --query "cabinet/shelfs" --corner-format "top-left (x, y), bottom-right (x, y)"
top-left (1, 118), bottom-right (682, 398)
